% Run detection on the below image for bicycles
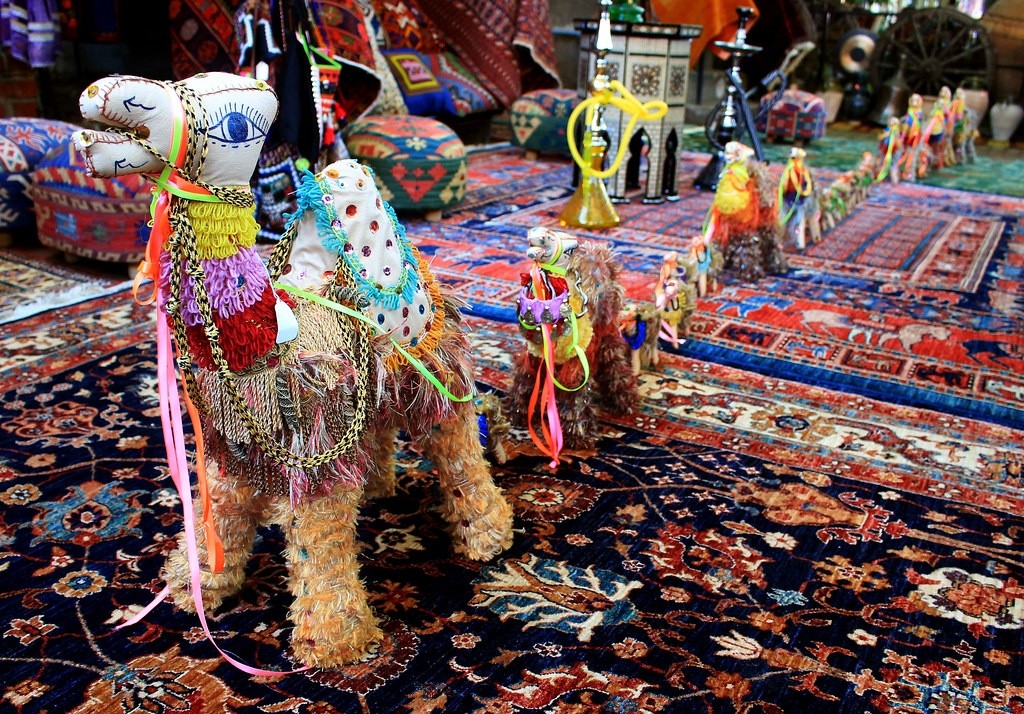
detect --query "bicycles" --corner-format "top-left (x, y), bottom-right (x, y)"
top-left (801, 0), bottom-right (997, 130)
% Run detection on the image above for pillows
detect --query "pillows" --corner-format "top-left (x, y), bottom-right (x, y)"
top-left (419, 52), bottom-right (499, 116)
top-left (383, 47), bottom-right (453, 115)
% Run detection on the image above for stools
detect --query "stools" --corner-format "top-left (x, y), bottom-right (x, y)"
top-left (344, 115), bottom-right (465, 221)
top-left (34, 137), bottom-right (160, 277)
top-left (0, 116), bottom-right (86, 246)
top-left (511, 89), bottom-right (586, 162)
top-left (761, 90), bottom-right (826, 146)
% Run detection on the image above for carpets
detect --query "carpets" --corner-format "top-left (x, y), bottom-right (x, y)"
top-left (0, 0), bottom-right (1024, 714)
